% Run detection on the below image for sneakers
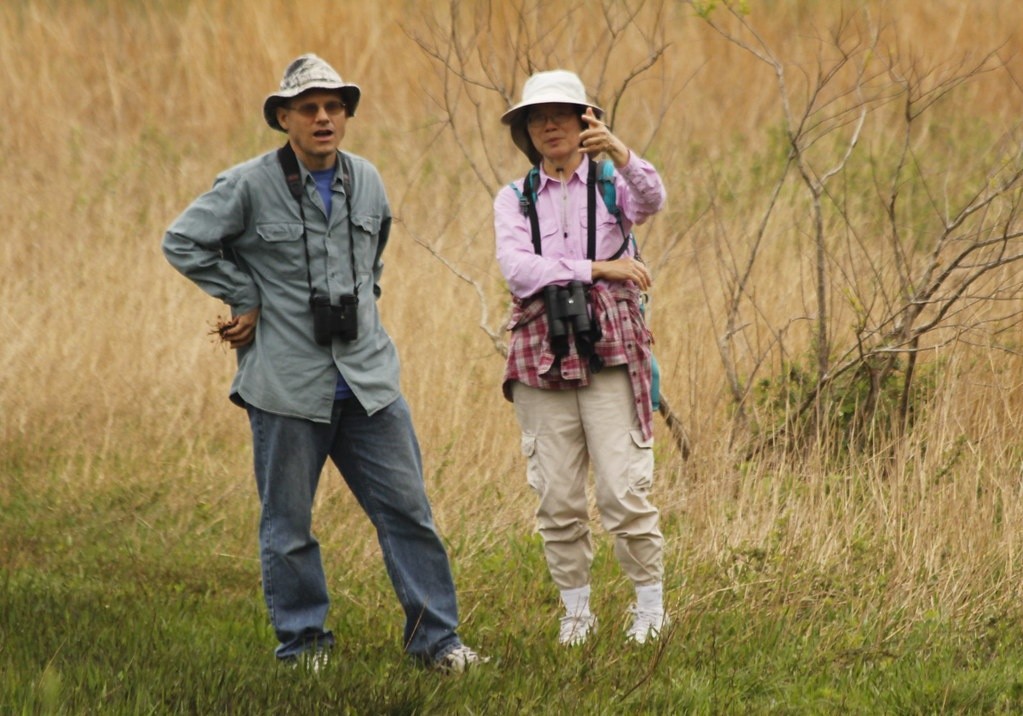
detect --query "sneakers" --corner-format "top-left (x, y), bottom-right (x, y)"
top-left (559, 615), bottom-right (598, 647)
top-left (433, 646), bottom-right (489, 675)
top-left (625, 607), bottom-right (671, 645)
top-left (287, 650), bottom-right (332, 678)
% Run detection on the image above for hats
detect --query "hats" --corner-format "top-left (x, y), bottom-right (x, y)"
top-left (501, 70), bottom-right (604, 128)
top-left (264, 54), bottom-right (362, 133)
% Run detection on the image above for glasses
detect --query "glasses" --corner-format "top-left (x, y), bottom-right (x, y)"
top-left (524, 111), bottom-right (580, 128)
top-left (278, 101), bottom-right (348, 118)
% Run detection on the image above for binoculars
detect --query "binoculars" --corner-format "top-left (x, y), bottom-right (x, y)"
top-left (311, 294), bottom-right (359, 345)
top-left (544, 280), bottom-right (591, 341)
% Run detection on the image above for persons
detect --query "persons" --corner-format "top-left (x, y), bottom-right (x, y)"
top-left (160, 52), bottom-right (491, 678)
top-left (492, 70), bottom-right (674, 647)
